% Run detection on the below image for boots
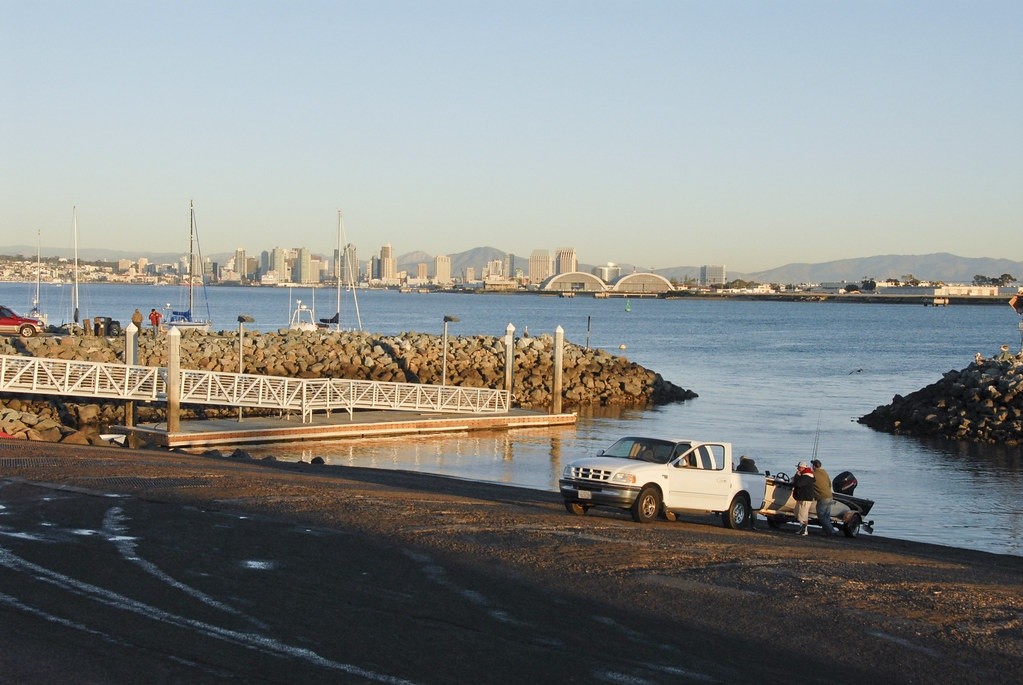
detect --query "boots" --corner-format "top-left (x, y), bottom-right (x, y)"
top-left (796, 525), bottom-right (808, 536)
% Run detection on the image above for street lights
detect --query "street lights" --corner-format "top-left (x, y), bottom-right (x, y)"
top-left (238, 315), bottom-right (256, 373)
top-left (442, 315), bottom-right (461, 405)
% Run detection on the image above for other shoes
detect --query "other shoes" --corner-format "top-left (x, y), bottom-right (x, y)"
top-left (822, 532), bottom-right (833, 537)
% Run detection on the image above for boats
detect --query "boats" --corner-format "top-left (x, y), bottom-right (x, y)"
top-left (288, 286), bottom-right (317, 332)
top-left (758, 470), bottom-right (875, 524)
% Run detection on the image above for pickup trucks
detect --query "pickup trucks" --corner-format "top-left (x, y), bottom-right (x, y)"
top-left (559, 436), bottom-right (766, 530)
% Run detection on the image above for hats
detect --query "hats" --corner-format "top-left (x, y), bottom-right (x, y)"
top-left (810, 460), bottom-right (821, 468)
top-left (152, 309), bottom-right (155, 312)
top-left (795, 461), bottom-right (807, 468)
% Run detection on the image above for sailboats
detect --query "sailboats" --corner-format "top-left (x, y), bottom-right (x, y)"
top-left (314, 210), bottom-right (363, 332)
top-left (60, 206), bottom-right (84, 329)
top-left (160, 199), bottom-right (212, 333)
top-left (22, 228), bottom-right (47, 325)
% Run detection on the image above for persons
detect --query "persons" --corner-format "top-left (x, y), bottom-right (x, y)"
top-left (811, 460), bottom-right (839, 538)
top-left (132, 308), bottom-right (143, 336)
top-left (736, 456), bottom-right (759, 530)
top-left (149, 309), bottom-right (162, 337)
top-left (793, 462), bottom-right (816, 536)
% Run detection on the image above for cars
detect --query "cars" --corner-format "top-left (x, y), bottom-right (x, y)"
top-left (0, 306), bottom-right (44, 338)
